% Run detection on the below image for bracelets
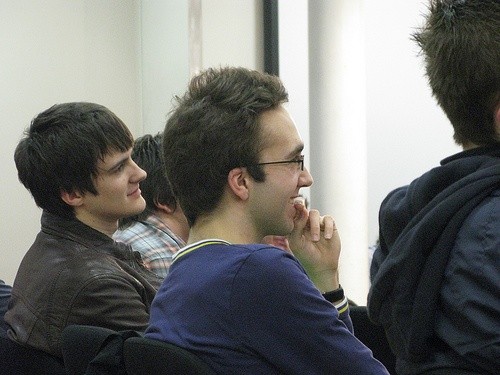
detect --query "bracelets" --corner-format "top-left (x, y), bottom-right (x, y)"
top-left (317, 284), bottom-right (343, 304)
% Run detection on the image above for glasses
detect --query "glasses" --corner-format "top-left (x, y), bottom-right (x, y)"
top-left (239, 153), bottom-right (305, 172)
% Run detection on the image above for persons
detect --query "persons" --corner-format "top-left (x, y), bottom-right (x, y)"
top-left (3, 102), bottom-right (167, 354)
top-left (112, 131), bottom-right (191, 286)
top-left (144, 67), bottom-right (390, 375)
top-left (367, 0), bottom-right (500, 375)
